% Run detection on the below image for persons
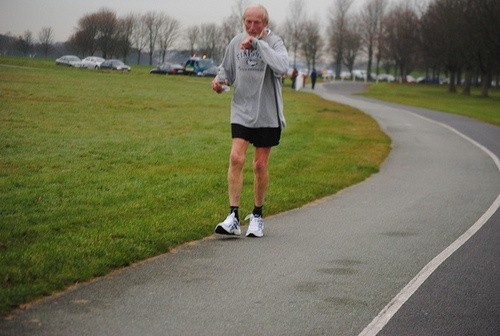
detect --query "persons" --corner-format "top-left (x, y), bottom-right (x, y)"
top-left (212, 5), bottom-right (288, 237)
top-left (301, 74), bottom-right (306, 87)
top-left (311, 68), bottom-right (316, 90)
top-left (291, 68), bottom-right (298, 88)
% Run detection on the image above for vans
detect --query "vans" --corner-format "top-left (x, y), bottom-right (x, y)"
top-left (184, 56), bottom-right (215, 76)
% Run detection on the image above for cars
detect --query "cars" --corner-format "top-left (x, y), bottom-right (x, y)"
top-left (55, 55), bottom-right (83, 69)
top-left (81, 56), bottom-right (105, 71)
top-left (203, 67), bottom-right (218, 76)
top-left (323, 69), bottom-right (482, 86)
top-left (150, 63), bottom-right (185, 76)
top-left (99, 58), bottom-right (132, 72)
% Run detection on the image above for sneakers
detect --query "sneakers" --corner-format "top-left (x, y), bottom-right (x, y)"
top-left (244, 213), bottom-right (264, 237)
top-left (214, 211), bottom-right (241, 236)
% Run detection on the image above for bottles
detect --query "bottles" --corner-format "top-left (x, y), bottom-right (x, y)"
top-left (216, 68), bottom-right (226, 93)
